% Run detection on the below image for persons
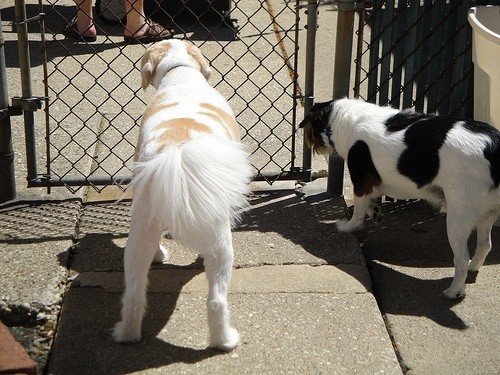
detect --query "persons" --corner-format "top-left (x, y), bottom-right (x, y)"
top-left (63, 0), bottom-right (175, 43)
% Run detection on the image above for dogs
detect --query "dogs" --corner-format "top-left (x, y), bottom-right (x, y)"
top-left (107, 38), bottom-right (252, 352)
top-left (299, 95), bottom-right (500, 302)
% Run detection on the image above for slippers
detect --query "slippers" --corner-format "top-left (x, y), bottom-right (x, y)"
top-left (62, 15), bottom-right (98, 42)
top-left (124, 17), bottom-right (175, 43)
